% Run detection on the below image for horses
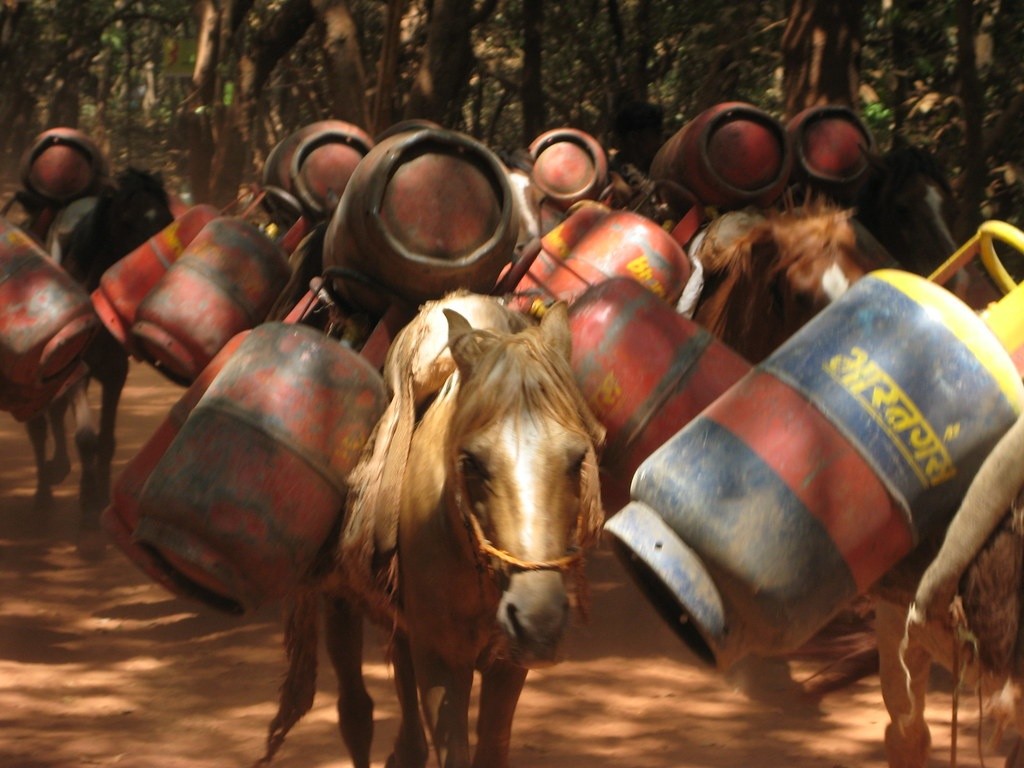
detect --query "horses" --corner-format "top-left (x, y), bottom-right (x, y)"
top-left (0, 83), bottom-right (1024, 768)
